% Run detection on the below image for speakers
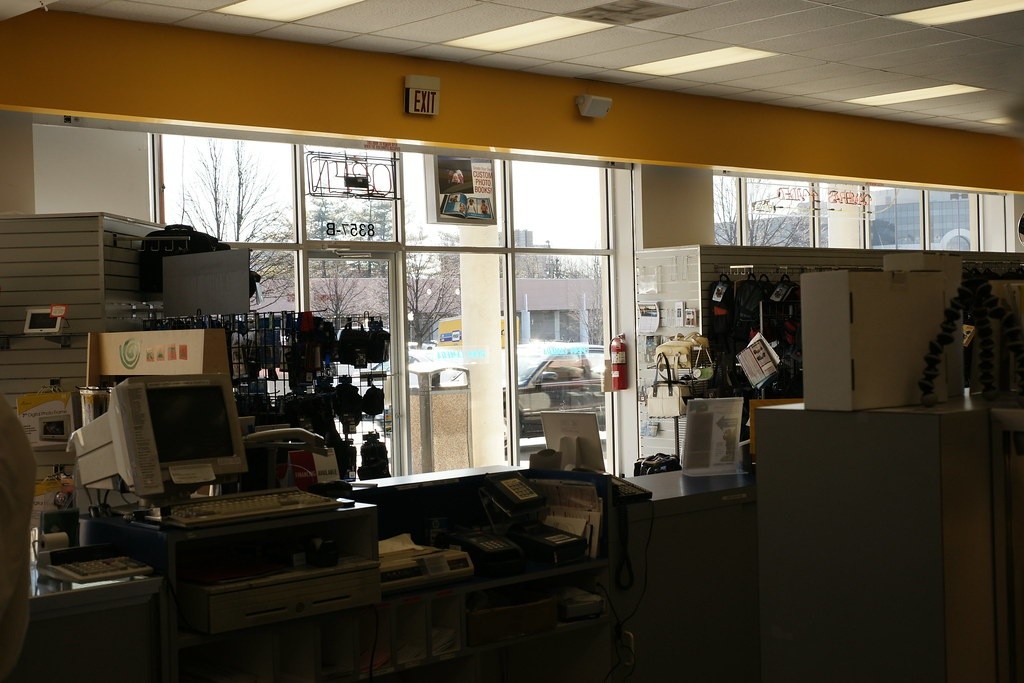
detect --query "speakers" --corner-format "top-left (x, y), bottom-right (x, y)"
top-left (578, 95), bottom-right (612, 119)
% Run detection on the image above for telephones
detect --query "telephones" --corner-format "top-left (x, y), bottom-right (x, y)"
top-left (573, 468), bottom-right (652, 505)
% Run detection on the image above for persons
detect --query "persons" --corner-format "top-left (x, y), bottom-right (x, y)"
top-left (448, 168), bottom-right (488, 214)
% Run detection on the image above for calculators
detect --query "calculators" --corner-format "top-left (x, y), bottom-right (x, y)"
top-left (33, 542), bottom-right (153, 584)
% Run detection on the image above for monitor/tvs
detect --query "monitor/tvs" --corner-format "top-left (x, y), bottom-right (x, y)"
top-left (72, 372), bottom-right (248, 508)
top-left (540, 411), bottom-right (606, 473)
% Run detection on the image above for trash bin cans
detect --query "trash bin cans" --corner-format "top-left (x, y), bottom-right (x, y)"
top-left (407, 360), bottom-right (472, 475)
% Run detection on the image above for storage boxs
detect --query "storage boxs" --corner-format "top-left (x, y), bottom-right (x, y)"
top-left (883, 252), bottom-right (964, 396)
top-left (800, 267), bottom-right (947, 411)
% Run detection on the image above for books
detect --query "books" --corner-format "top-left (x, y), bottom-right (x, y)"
top-left (531, 478), bottom-right (602, 561)
top-left (439, 193), bottom-right (494, 220)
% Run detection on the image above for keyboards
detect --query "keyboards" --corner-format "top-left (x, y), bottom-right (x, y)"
top-left (163, 486), bottom-right (342, 527)
top-left (609, 475), bottom-right (652, 503)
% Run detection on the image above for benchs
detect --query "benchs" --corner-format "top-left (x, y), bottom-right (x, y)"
top-left (527, 366), bottom-right (584, 382)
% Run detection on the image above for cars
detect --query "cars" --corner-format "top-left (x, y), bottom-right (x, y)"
top-left (405, 341), bottom-right (606, 449)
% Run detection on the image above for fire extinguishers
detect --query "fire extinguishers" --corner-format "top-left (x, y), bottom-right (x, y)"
top-left (609, 333), bottom-right (629, 390)
